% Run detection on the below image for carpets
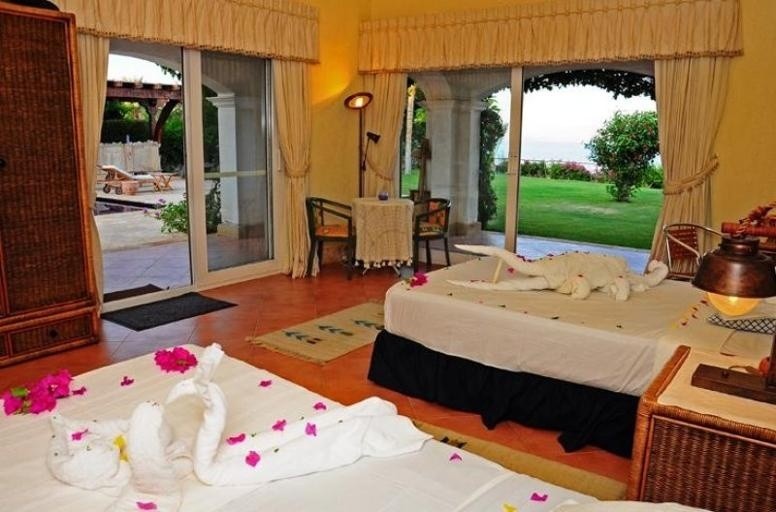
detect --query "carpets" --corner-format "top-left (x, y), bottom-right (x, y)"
top-left (245, 298), bottom-right (385, 366)
top-left (100, 291), bottom-right (240, 332)
top-left (411, 419), bottom-right (629, 501)
top-left (103, 282), bottom-right (170, 303)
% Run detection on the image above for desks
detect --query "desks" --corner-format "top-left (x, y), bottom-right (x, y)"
top-left (352, 198), bottom-right (415, 277)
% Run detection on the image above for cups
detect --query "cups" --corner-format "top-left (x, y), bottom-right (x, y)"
top-left (378, 192), bottom-right (389, 200)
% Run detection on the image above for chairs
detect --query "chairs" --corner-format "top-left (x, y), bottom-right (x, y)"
top-left (305, 198), bottom-right (355, 282)
top-left (95, 163), bottom-right (180, 195)
top-left (410, 196), bottom-right (452, 272)
top-left (662, 222), bottom-right (726, 282)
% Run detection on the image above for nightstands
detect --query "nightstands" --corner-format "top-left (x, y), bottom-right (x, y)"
top-left (624, 342), bottom-right (776, 511)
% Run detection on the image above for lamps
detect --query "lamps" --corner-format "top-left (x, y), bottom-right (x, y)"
top-left (344, 92), bottom-right (382, 197)
top-left (688, 233), bottom-right (776, 406)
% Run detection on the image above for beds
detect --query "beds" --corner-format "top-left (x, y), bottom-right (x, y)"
top-left (0, 344), bottom-right (687, 510)
